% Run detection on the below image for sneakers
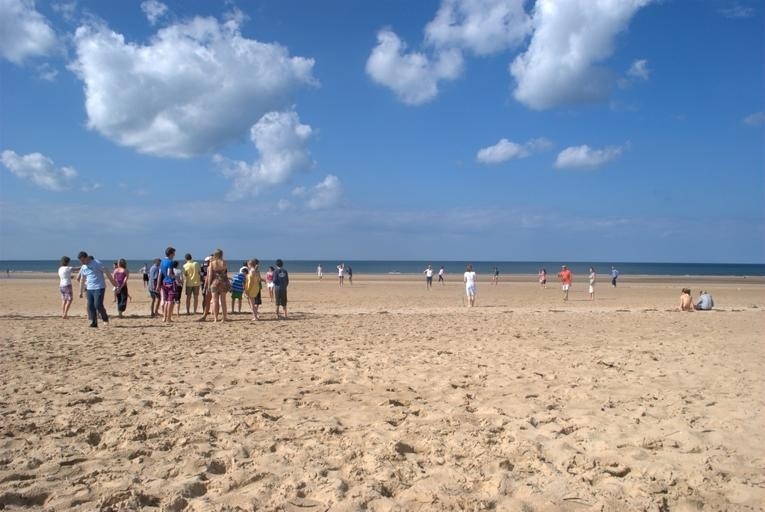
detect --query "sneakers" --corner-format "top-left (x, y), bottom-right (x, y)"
top-left (90, 317), bottom-right (109, 327)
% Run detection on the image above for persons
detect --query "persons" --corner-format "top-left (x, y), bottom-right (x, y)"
top-left (494, 267), bottom-right (499, 283)
top-left (560, 265), bottom-right (572, 300)
top-left (438, 266), bottom-right (444, 281)
top-left (336, 264), bottom-right (344, 287)
top-left (345, 264), bottom-right (352, 286)
top-left (58, 246), bottom-right (289, 327)
top-left (463, 264), bottom-right (477, 306)
top-left (681, 287), bottom-right (713, 310)
top-left (588, 267), bottom-right (595, 300)
top-left (611, 265), bottom-right (618, 286)
top-left (539, 264), bottom-right (547, 288)
top-left (424, 265), bottom-right (433, 288)
top-left (317, 264), bottom-right (323, 279)
